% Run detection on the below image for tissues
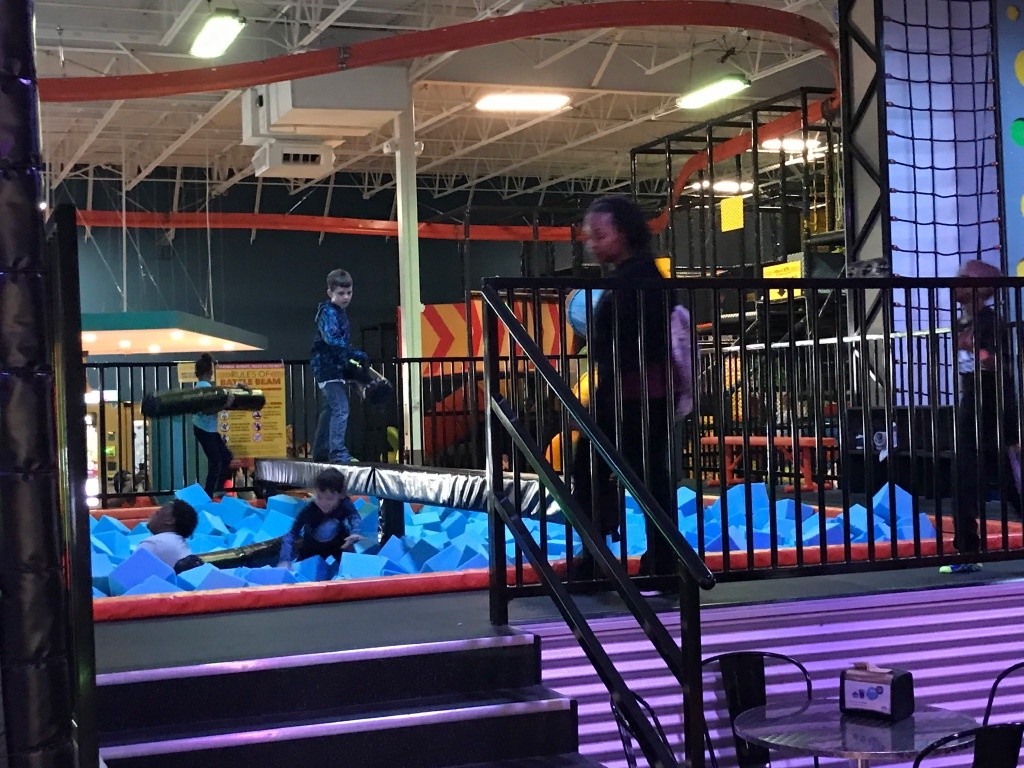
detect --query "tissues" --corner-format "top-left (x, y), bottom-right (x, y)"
top-left (838, 658), bottom-right (916, 721)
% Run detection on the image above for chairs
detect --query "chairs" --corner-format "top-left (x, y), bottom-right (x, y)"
top-left (611, 651), bottom-right (1024, 768)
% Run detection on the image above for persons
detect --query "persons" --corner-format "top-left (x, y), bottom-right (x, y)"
top-left (174, 467), bottom-right (366, 575)
top-left (502, 454), bottom-right (509, 471)
top-left (299, 439), bottom-right (313, 458)
top-left (939, 259), bottom-right (1024, 573)
top-left (134, 463), bottom-right (151, 491)
top-left (310, 268), bottom-right (368, 461)
top-left (193, 352), bottom-right (232, 499)
top-left (236, 458), bottom-right (256, 500)
top-left (133, 499), bottom-right (198, 568)
top-left (231, 466), bottom-right (243, 487)
top-left (554, 194), bottom-right (683, 595)
top-left (286, 424), bottom-right (303, 458)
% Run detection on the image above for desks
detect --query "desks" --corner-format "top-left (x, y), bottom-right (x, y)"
top-left (734, 699), bottom-right (982, 768)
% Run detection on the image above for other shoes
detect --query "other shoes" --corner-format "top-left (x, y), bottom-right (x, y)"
top-left (636, 558), bottom-right (672, 596)
top-left (330, 452), bottom-right (361, 463)
top-left (550, 552), bottom-right (621, 594)
